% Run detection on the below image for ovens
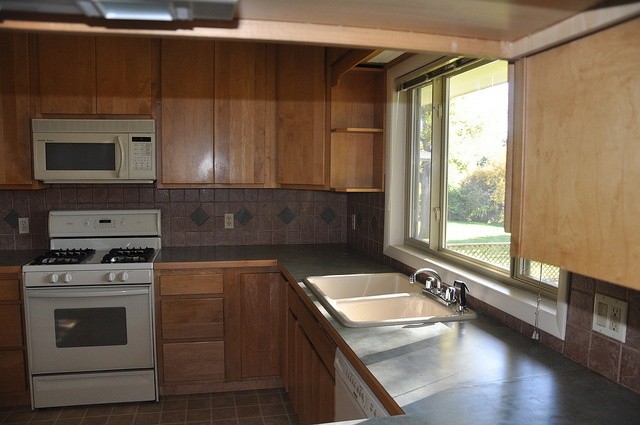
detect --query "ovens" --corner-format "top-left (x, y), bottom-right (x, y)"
top-left (23, 284), bottom-right (159, 411)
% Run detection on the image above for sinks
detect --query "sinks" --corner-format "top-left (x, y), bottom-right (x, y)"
top-left (304, 272), bottom-right (426, 299)
top-left (331, 295), bottom-right (479, 328)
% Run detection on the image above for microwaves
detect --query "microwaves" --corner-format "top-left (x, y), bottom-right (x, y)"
top-left (30, 117), bottom-right (157, 186)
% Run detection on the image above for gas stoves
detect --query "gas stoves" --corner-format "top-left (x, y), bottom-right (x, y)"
top-left (21, 238), bottom-right (162, 284)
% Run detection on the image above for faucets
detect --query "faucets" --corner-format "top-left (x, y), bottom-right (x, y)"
top-left (453, 280), bottom-right (470, 307)
top-left (409, 266), bottom-right (442, 290)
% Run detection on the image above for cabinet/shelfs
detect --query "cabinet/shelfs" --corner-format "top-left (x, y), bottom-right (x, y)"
top-left (1, 247), bottom-right (46, 408)
top-left (157, 35), bottom-right (276, 190)
top-left (280, 280), bottom-right (392, 424)
top-left (31, 31), bottom-right (153, 122)
top-left (1, 28), bottom-right (51, 192)
top-left (153, 265), bottom-right (289, 398)
top-left (276, 41), bottom-right (331, 191)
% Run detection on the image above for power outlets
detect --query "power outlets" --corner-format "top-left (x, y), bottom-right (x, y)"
top-left (350, 213), bottom-right (356, 230)
top-left (224, 213), bottom-right (234, 228)
top-left (18, 217), bottom-right (29, 234)
